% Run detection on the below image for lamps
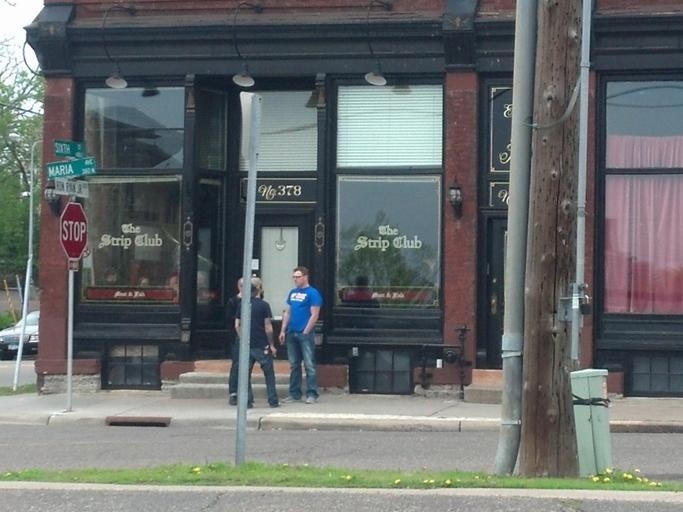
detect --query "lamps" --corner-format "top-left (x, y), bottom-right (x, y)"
top-left (358, 1), bottom-right (393, 87)
top-left (97, 4), bottom-right (138, 91)
top-left (228, 1), bottom-right (267, 90)
top-left (446, 176), bottom-right (465, 218)
top-left (41, 175), bottom-right (63, 217)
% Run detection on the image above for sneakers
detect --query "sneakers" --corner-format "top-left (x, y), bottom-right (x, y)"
top-left (306, 396), bottom-right (319, 403)
top-left (230, 396), bottom-right (237, 405)
top-left (282, 396), bottom-right (301, 403)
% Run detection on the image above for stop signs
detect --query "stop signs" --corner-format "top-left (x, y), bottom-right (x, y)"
top-left (58, 200), bottom-right (87, 261)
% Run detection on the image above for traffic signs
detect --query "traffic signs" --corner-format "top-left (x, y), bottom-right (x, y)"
top-left (45, 156), bottom-right (98, 180)
top-left (52, 176), bottom-right (90, 199)
top-left (53, 139), bottom-right (85, 158)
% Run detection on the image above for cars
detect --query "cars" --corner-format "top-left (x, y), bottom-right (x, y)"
top-left (0, 309), bottom-right (39, 362)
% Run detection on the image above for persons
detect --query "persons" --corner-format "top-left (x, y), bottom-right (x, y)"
top-left (278, 266), bottom-right (325, 403)
top-left (341, 275), bottom-right (372, 301)
top-left (233, 277), bottom-right (280, 408)
top-left (163, 272), bottom-right (178, 287)
top-left (98, 267), bottom-right (120, 285)
top-left (226, 273), bottom-right (264, 408)
top-left (402, 262), bottom-right (436, 303)
